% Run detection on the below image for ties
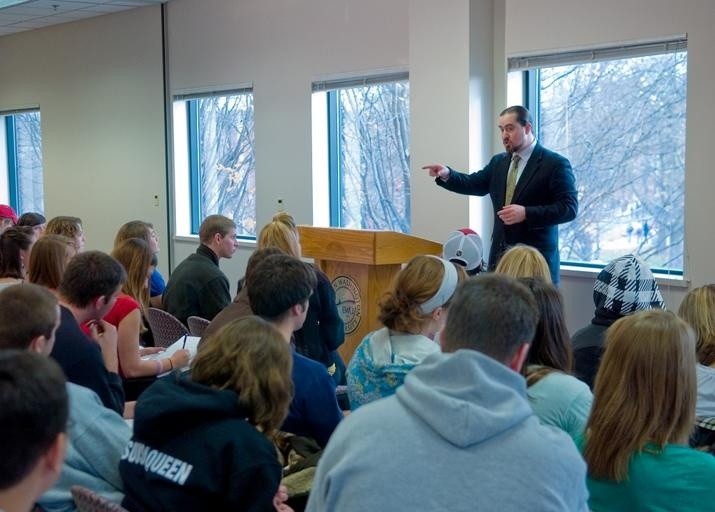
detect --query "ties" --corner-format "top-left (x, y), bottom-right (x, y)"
top-left (505, 155), bottom-right (522, 206)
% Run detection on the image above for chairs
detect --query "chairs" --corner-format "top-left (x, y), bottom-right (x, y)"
top-left (68, 485), bottom-right (131, 512)
top-left (144, 306), bottom-right (186, 348)
top-left (186, 317), bottom-right (211, 336)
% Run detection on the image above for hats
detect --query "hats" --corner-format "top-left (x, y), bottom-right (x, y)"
top-left (0, 204), bottom-right (17, 225)
top-left (443, 228), bottom-right (484, 270)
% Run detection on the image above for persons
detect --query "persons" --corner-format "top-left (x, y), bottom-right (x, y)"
top-left (678, 283), bottom-right (714, 418)
top-left (303, 270), bottom-right (590, 512)
top-left (519, 277), bottom-right (594, 455)
top-left (567, 252), bottom-right (667, 395)
top-left (494, 244), bottom-right (554, 284)
top-left (421, 105), bottom-right (579, 291)
top-left (0, 205), bottom-right (347, 510)
top-left (427, 227), bottom-right (486, 343)
top-left (574, 309), bottom-right (715, 512)
top-left (345, 252), bottom-right (470, 413)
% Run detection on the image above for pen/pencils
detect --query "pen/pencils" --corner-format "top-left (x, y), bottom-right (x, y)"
top-left (183, 334), bottom-right (188, 349)
